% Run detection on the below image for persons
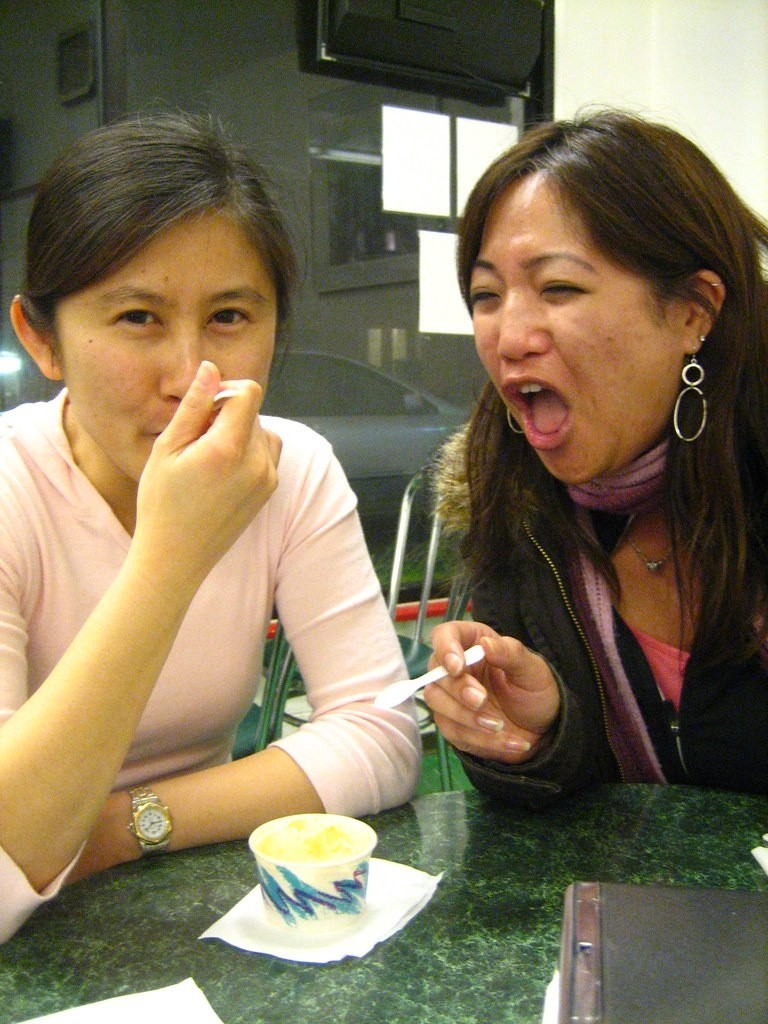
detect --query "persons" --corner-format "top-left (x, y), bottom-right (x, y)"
top-left (425, 111), bottom-right (766, 806)
top-left (0, 110), bottom-right (420, 943)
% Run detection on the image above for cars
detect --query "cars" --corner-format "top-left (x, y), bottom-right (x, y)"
top-left (261, 348), bottom-right (482, 557)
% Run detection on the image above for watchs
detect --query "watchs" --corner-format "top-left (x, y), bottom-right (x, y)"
top-left (125, 784), bottom-right (172, 858)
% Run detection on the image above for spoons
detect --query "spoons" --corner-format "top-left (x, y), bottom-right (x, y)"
top-left (375, 646), bottom-right (485, 710)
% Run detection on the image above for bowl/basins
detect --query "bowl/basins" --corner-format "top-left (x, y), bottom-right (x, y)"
top-left (244, 814), bottom-right (379, 939)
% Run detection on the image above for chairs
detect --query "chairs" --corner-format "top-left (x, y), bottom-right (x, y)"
top-left (231, 468), bottom-right (474, 793)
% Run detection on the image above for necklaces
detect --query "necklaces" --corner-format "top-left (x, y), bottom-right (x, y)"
top-left (626, 518), bottom-right (687, 572)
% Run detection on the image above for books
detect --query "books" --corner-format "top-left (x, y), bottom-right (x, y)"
top-left (562, 879), bottom-right (768, 1024)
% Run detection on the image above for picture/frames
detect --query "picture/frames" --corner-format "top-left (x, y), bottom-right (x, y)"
top-left (51, 21), bottom-right (95, 105)
top-left (307, 82), bottom-right (440, 294)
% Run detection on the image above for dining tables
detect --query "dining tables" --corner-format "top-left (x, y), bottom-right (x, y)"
top-left (1, 784), bottom-right (768, 1024)
